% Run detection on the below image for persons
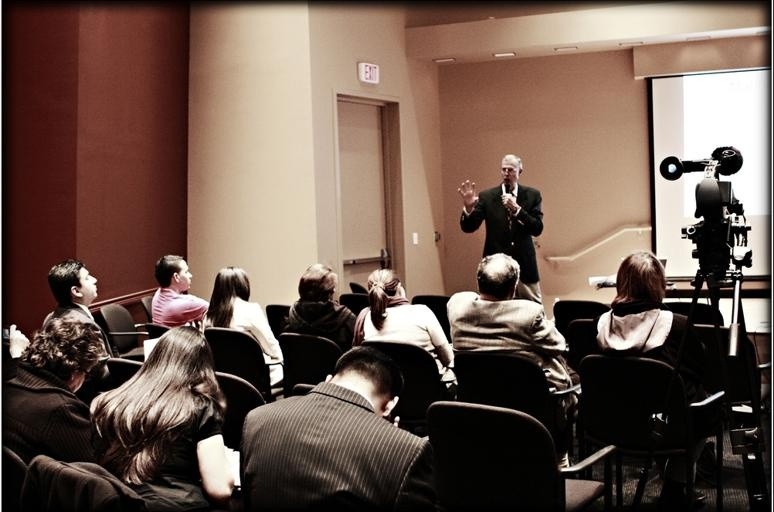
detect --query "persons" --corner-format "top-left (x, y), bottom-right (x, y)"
top-left (201, 266), bottom-right (284, 401)
top-left (445, 251), bottom-right (582, 467)
top-left (588, 251), bottom-right (711, 504)
top-left (456, 153), bottom-right (544, 304)
top-left (284, 263), bottom-right (357, 355)
top-left (88, 325), bottom-right (240, 511)
top-left (151, 254), bottom-right (210, 329)
top-left (2, 317), bottom-right (97, 470)
top-left (350, 269), bottom-right (458, 400)
top-left (239, 346), bottom-right (442, 511)
top-left (41, 259), bottom-right (114, 407)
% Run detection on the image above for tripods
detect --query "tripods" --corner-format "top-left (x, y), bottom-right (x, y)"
top-left (630, 269), bottom-right (771, 512)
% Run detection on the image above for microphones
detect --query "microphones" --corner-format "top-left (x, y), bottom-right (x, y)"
top-left (505, 183), bottom-right (510, 211)
